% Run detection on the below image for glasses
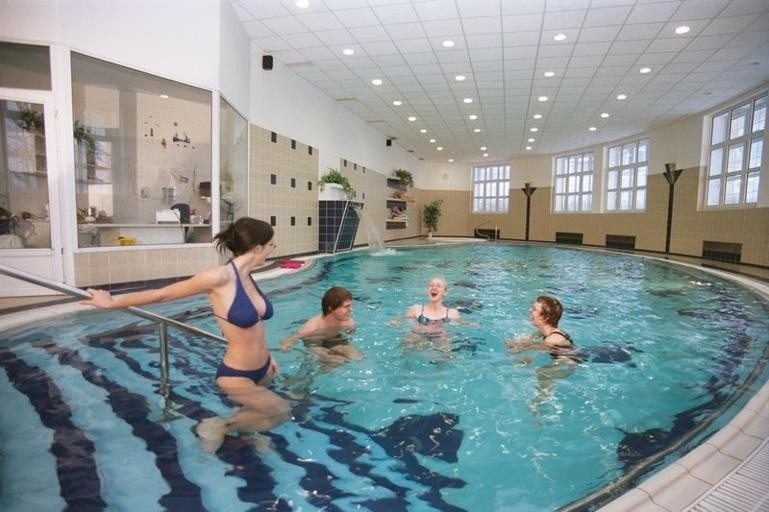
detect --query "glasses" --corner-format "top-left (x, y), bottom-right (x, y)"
top-left (263, 241), bottom-right (277, 251)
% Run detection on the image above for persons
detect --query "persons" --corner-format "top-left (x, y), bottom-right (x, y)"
top-left (503, 296), bottom-right (579, 430)
top-left (79, 218), bottom-right (293, 454)
top-left (390, 277), bottom-right (479, 364)
top-left (278, 286), bottom-right (363, 373)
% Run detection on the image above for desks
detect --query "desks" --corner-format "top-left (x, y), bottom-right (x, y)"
top-left (84, 223), bottom-right (212, 246)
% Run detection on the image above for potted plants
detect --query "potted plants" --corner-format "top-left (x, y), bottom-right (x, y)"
top-left (316, 169), bottom-right (352, 201)
top-left (424, 197), bottom-right (444, 237)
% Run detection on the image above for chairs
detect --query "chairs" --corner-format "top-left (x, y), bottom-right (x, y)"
top-left (169, 202), bottom-right (191, 236)
